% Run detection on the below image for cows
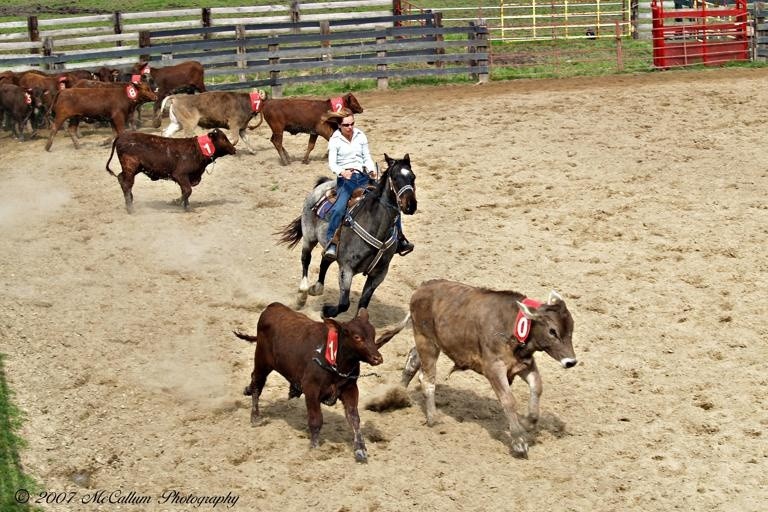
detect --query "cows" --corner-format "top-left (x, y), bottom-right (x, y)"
top-left (232, 301), bottom-right (386, 467)
top-left (105, 130), bottom-right (238, 213)
top-left (1, 58), bottom-right (206, 151)
top-left (156, 89), bottom-right (270, 158)
top-left (375, 278), bottom-right (578, 459)
top-left (245, 93), bottom-right (364, 167)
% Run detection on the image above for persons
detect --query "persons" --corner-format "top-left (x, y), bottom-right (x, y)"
top-left (316, 107), bottom-right (414, 259)
top-left (584, 26), bottom-right (594, 39)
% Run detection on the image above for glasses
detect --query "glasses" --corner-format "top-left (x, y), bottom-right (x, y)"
top-left (338, 122), bottom-right (355, 127)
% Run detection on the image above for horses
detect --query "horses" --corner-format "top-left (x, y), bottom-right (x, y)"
top-left (270, 152), bottom-right (420, 319)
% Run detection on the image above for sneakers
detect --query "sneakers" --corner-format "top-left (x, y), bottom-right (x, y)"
top-left (324, 243), bottom-right (337, 258)
top-left (395, 241), bottom-right (415, 253)
